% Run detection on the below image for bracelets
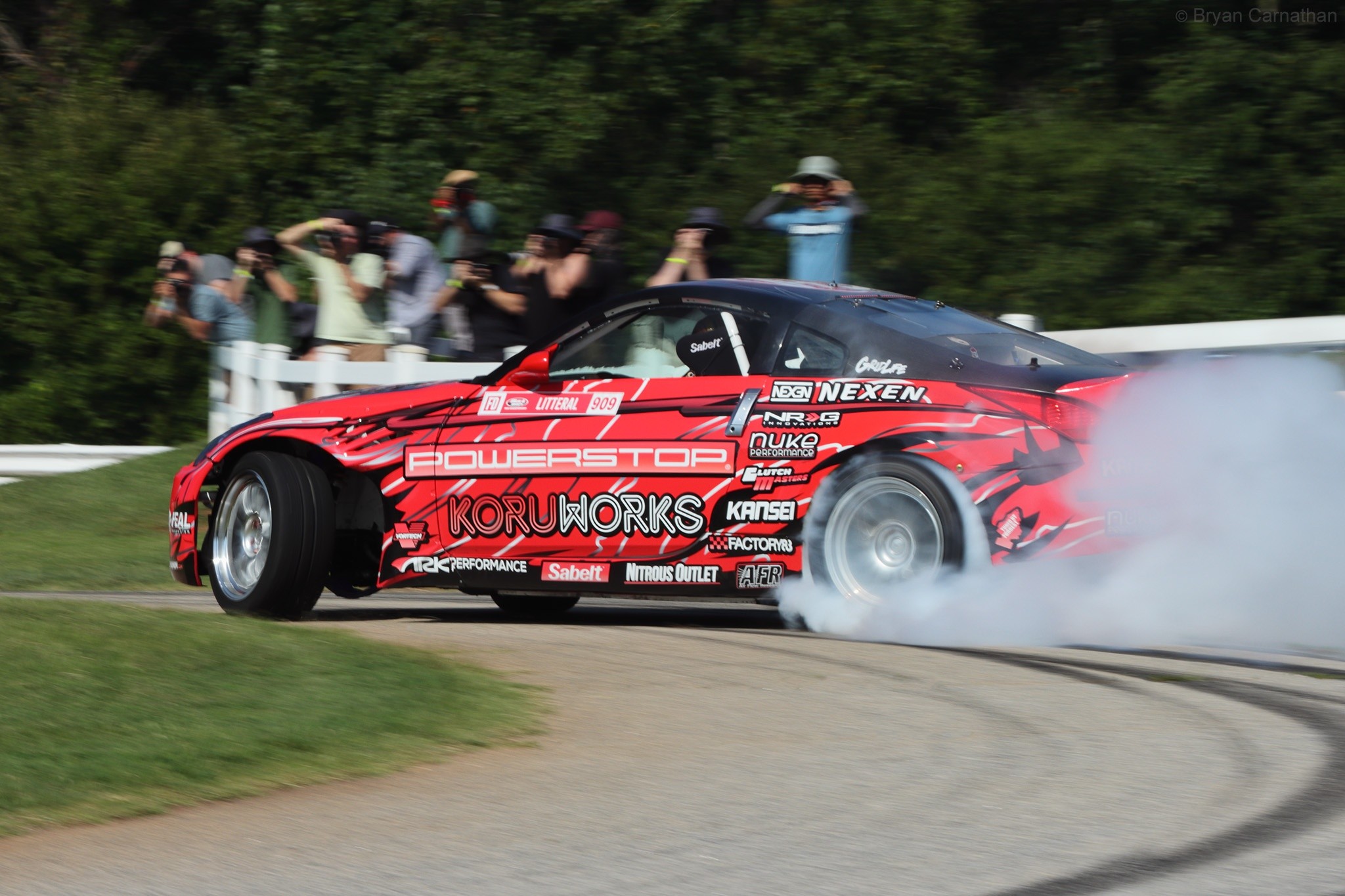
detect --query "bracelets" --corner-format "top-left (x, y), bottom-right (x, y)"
top-left (665, 257), bottom-right (689, 265)
top-left (481, 283), bottom-right (500, 290)
top-left (233, 268), bottom-right (250, 277)
top-left (444, 278), bottom-right (464, 288)
top-left (308, 218), bottom-right (323, 232)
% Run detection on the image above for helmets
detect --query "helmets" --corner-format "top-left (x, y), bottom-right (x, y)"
top-left (691, 315), bottom-right (755, 334)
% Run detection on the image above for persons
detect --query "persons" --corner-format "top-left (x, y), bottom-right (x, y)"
top-left (682, 314), bottom-right (747, 380)
top-left (741, 155), bottom-right (868, 283)
top-left (428, 168), bottom-right (500, 267)
top-left (138, 227), bottom-right (322, 359)
top-left (275, 214), bottom-right (445, 351)
top-left (429, 209), bottom-right (631, 363)
top-left (644, 206), bottom-right (732, 287)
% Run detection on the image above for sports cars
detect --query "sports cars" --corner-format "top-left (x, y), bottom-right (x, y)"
top-left (159, 273), bottom-right (1193, 626)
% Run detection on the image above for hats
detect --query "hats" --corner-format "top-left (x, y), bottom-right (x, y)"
top-left (576, 210), bottom-right (620, 231)
top-left (790, 155), bottom-right (845, 184)
top-left (685, 206), bottom-right (733, 234)
top-left (534, 212), bottom-right (581, 241)
top-left (158, 239), bottom-right (184, 258)
